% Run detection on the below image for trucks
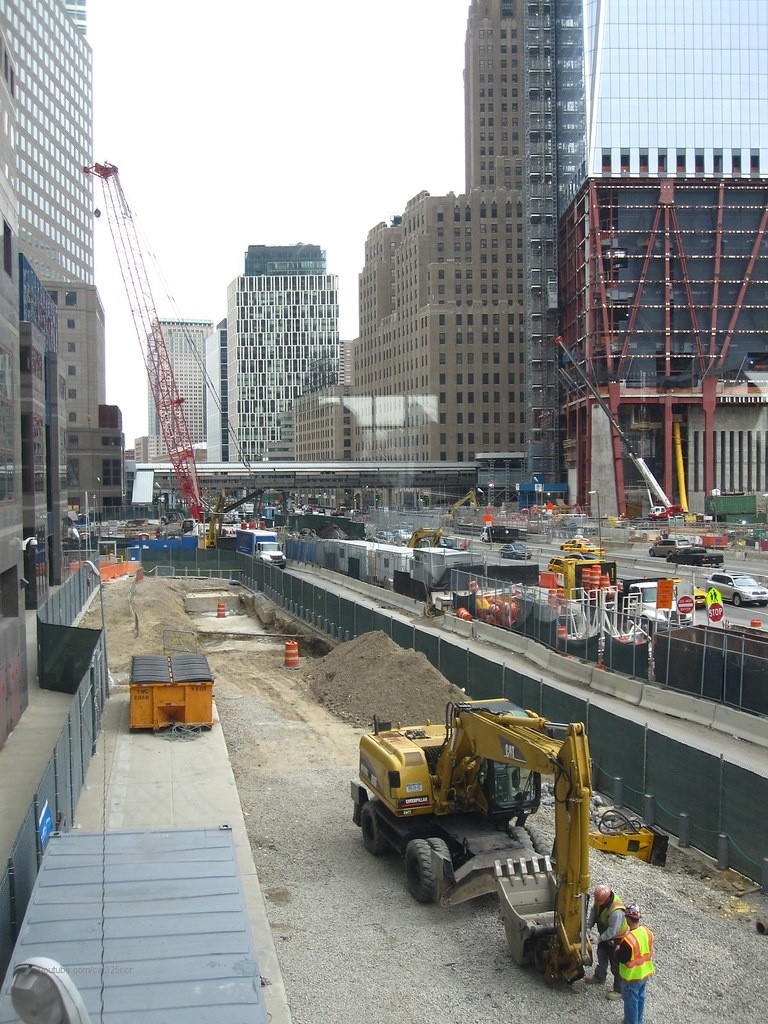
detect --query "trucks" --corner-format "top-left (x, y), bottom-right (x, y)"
top-left (618, 577), bottom-right (693, 638)
top-left (180, 518), bottom-right (243, 536)
top-left (480, 526), bottom-right (519, 544)
top-left (235, 528), bottom-right (289, 570)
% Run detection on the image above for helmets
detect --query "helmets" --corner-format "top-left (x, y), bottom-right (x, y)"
top-left (625, 904), bottom-right (641, 919)
top-left (594, 885), bottom-right (611, 905)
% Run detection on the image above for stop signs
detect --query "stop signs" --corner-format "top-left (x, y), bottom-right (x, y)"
top-left (707, 603), bottom-right (724, 622)
top-left (677, 595), bottom-right (695, 614)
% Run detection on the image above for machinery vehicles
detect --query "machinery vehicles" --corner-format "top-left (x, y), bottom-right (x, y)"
top-left (407, 526), bottom-right (443, 548)
top-left (442, 490), bottom-right (480, 527)
top-left (348, 697), bottom-right (593, 989)
top-left (200, 488), bottom-right (226, 549)
top-left (553, 334), bottom-right (684, 522)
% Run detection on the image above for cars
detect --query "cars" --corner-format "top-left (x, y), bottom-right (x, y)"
top-left (499, 543), bottom-right (532, 560)
top-left (296, 504), bottom-right (361, 517)
top-left (560, 535), bottom-right (590, 550)
top-left (668, 577), bottom-right (708, 609)
top-left (705, 572), bottom-right (768, 607)
top-left (564, 542), bottom-right (605, 555)
top-left (648, 538), bottom-right (692, 558)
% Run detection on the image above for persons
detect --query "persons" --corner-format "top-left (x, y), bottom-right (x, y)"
top-left (586, 885), bottom-right (630, 1001)
top-left (615, 904), bottom-right (655, 1024)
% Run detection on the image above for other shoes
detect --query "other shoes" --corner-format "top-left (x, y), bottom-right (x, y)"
top-left (605, 991), bottom-right (622, 1000)
top-left (586, 974), bottom-right (605, 983)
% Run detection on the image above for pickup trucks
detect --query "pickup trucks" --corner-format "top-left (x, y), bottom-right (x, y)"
top-left (665, 547), bottom-right (725, 569)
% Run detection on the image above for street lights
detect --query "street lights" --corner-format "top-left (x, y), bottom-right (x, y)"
top-left (588, 490), bottom-right (602, 557)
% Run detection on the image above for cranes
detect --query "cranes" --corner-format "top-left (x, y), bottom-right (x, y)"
top-left (85, 158), bottom-right (211, 523)
